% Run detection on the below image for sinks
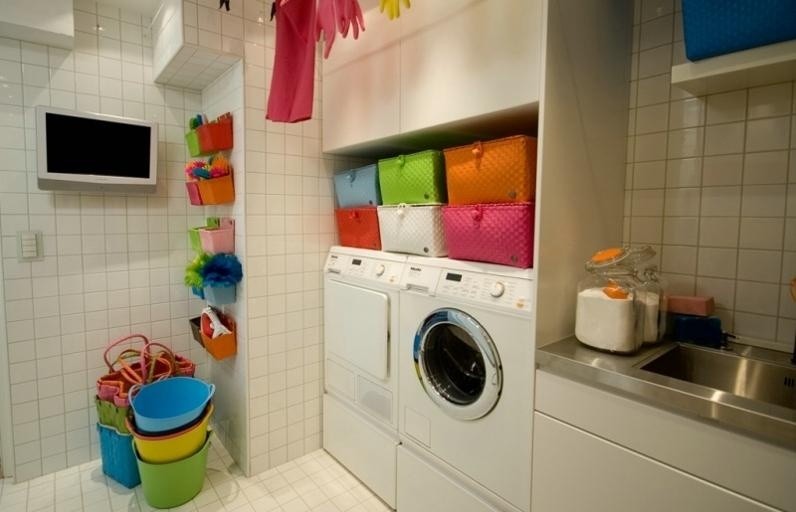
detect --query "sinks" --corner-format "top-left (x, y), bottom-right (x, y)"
top-left (636, 341), bottom-right (796, 419)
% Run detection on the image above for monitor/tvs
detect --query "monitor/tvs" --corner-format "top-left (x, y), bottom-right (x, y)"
top-left (35, 105), bottom-right (157, 194)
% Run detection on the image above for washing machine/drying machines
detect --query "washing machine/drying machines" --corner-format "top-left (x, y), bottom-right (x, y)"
top-left (397, 256), bottom-right (537, 511)
top-left (320, 245), bottom-right (405, 511)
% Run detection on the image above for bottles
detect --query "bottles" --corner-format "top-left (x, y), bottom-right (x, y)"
top-left (632, 246), bottom-right (669, 348)
top-left (575, 248), bottom-right (648, 355)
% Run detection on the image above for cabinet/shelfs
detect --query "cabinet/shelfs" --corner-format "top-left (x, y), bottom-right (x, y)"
top-left (320, 0), bottom-right (631, 278)
top-left (529, 367), bottom-right (796, 511)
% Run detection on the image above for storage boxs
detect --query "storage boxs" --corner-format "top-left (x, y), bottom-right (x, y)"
top-left (331, 134), bottom-right (535, 271)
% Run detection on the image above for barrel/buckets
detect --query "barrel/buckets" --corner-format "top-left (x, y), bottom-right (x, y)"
top-left (124, 378), bottom-right (216, 510)
